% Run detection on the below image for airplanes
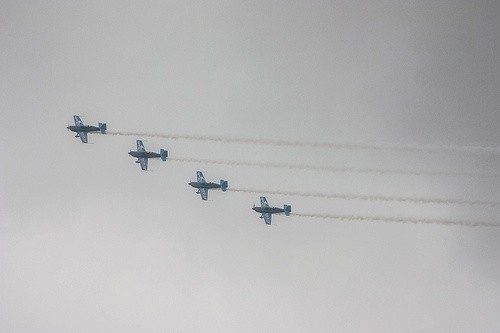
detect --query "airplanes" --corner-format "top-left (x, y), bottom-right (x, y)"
top-left (127, 139), bottom-right (168, 170)
top-left (188, 170), bottom-right (227, 199)
top-left (66, 115), bottom-right (106, 143)
top-left (251, 197), bottom-right (291, 225)
